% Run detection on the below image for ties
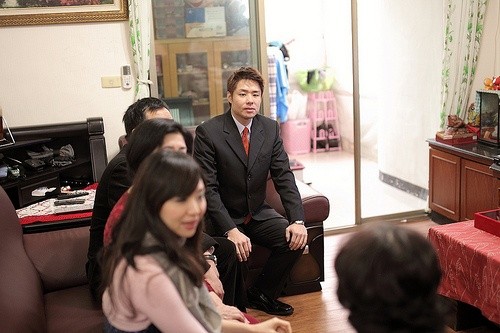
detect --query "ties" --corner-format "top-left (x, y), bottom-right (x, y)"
top-left (241, 127), bottom-right (251, 225)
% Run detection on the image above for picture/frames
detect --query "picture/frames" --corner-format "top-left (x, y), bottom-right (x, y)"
top-left (0, 0), bottom-right (129, 27)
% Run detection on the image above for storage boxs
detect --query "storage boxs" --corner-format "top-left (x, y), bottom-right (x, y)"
top-left (279, 119), bottom-right (311, 155)
top-left (436, 134), bottom-right (477, 145)
top-left (474, 209), bottom-right (500, 237)
top-left (476, 91), bottom-right (500, 147)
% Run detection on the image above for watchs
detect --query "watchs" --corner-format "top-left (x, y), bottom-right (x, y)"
top-left (294, 219), bottom-right (306, 225)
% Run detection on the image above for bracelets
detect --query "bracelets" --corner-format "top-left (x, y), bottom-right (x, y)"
top-left (202, 253), bottom-right (219, 267)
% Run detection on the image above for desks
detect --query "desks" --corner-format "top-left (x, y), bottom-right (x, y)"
top-left (427, 221), bottom-right (500, 330)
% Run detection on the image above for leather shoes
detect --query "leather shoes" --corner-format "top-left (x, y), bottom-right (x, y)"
top-left (222, 296), bottom-right (247, 313)
top-left (245, 293), bottom-right (293, 315)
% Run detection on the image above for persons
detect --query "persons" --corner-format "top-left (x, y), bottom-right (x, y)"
top-left (193, 64), bottom-right (308, 317)
top-left (332, 216), bottom-right (446, 333)
top-left (83, 93), bottom-right (293, 333)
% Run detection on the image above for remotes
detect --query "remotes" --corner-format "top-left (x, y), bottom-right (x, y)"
top-left (54, 199), bottom-right (85, 206)
top-left (57, 192), bottom-right (90, 200)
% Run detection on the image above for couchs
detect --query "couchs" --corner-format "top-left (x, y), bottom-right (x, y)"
top-left (0, 174), bottom-right (330, 333)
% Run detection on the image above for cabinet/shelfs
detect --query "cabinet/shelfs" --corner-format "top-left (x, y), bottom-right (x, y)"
top-left (0, 119), bottom-right (107, 209)
top-left (426, 139), bottom-right (500, 221)
top-left (309, 97), bottom-right (342, 153)
